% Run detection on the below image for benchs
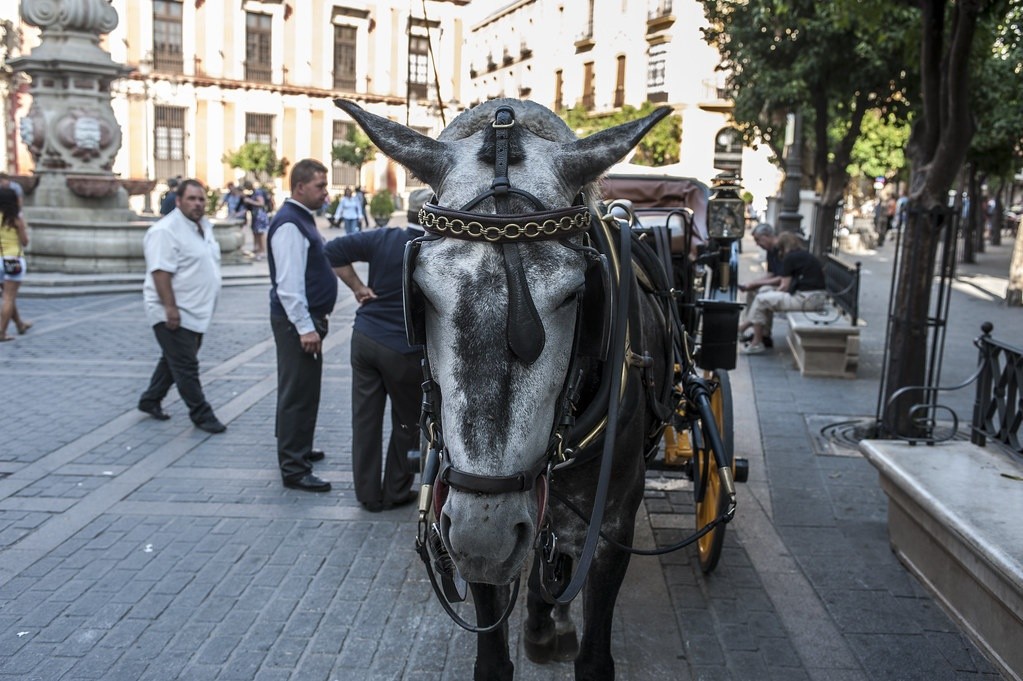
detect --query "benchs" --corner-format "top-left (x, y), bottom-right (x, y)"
top-left (786, 246), bottom-right (867, 379)
top-left (595, 176), bottom-right (712, 262)
top-left (858, 322), bottom-right (1023, 681)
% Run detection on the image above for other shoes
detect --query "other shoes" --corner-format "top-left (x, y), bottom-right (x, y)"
top-left (137, 402), bottom-right (170, 420)
top-left (195, 420), bottom-right (226, 432)
top-left (363, 501), bottom-right (382, 512)
top-left (18, 320), bottom-right (32, 334)
top-left (737, 341), bottom-right (766, 354)
top-left (0, 335), bottom-right (15, 341)
top-left (384, 490), bottom-right (419, 509)
top-left (740, 334), bottom-right (773, 347)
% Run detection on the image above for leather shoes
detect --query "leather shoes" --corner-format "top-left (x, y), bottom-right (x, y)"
top-left (309, 449), bottom-right (324, 461)
top-left (283, 473), bottom-right (331, 492)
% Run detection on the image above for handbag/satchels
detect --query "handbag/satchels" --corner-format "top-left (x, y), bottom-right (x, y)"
top-left (3, 260), bottom-right (22, 273)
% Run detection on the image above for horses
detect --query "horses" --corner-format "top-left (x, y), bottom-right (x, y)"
top-left (333, 91), bottom-right (675, 681)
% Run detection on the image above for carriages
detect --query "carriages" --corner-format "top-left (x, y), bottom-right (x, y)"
top-left (333, 97), bottom-right (749, 681)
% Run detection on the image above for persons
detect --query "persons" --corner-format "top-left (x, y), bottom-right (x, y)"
top-left (840, 190), bottom-right (996, 239)
top-left (746, 200), bottom-right (760, 229)
top-left (317, 185), bottom-right (369, 234)
top-left (738, 223), bottom-right (827, 354)
top-left (0, 171), bottom-right (33, 342)
top-left (158, 176), bottom-right (183, 216)
top-left (267, 158), bottom-right (338, 492)
top-left (136, 179), bottom-right (227, 434)
top-left (220, 180), bottom-right (270, 259)
top-left (325, 188), bottom-right (434, 511)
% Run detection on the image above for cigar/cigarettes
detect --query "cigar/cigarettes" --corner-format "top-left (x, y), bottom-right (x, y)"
top-left (314, 351), bottom-right (317, 360)
